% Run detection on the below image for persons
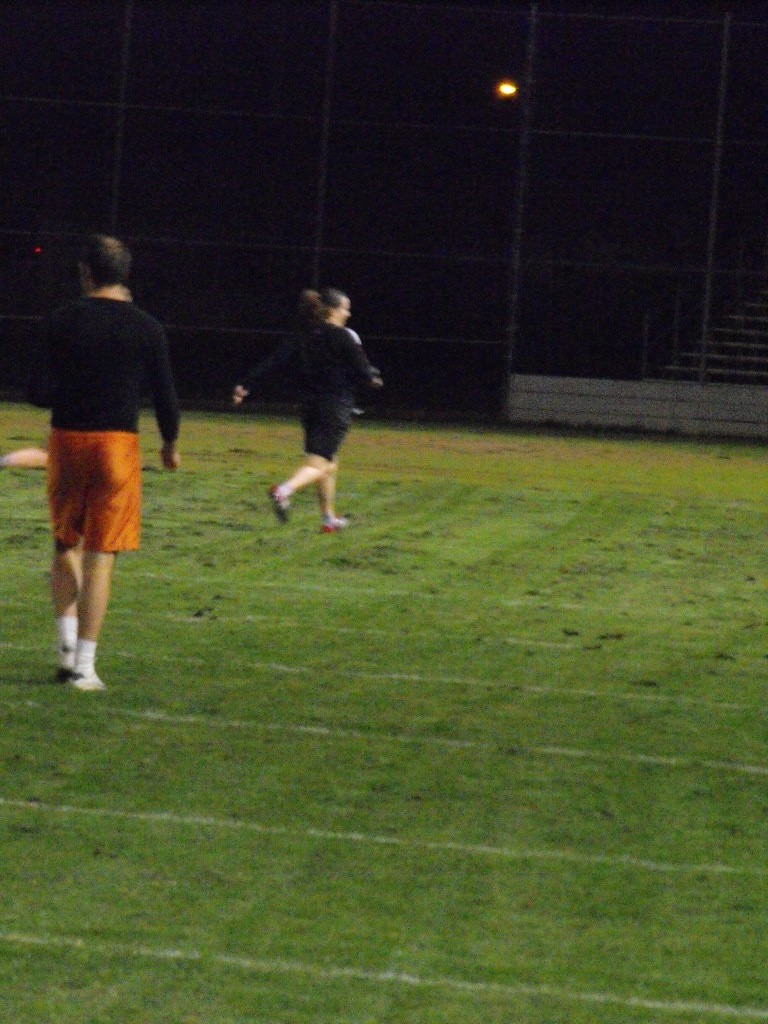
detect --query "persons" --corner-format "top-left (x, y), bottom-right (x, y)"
top-left (232, 289), bottom-right (383, 531)
top-left (31, 235), bottom-right (179, 689)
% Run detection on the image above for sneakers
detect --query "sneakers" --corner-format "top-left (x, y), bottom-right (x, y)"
top-left (67, 671), bottom-right (107, 692)
top-left (269, 483), bottom-right (293, 524)
top-left (319, 514), bottom-right (351, 534)
top-left (55, 640), bottom-right (77, 672)
